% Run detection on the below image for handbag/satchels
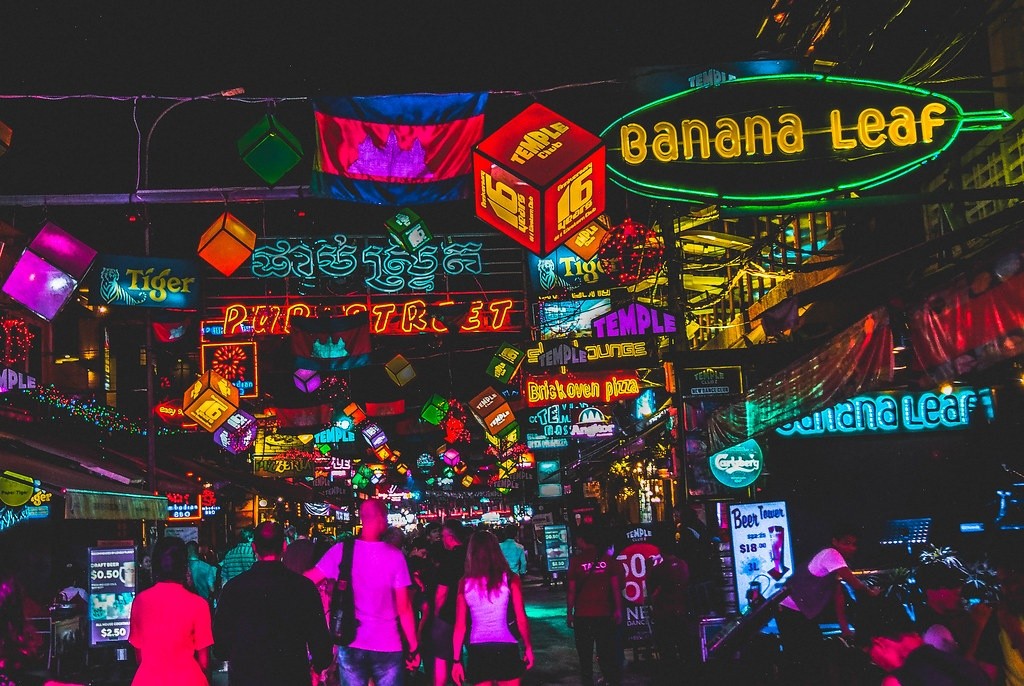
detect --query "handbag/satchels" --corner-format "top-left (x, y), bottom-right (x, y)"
top-left (329, 538), bottom-right (361, 644)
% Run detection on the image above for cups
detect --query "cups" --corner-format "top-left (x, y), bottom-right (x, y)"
top-left (91, 592), bottom-right (135, 619)
top-left (768, 525), bottom-right (788, 579)
top-left (119, 562), bottom-right (136, 588)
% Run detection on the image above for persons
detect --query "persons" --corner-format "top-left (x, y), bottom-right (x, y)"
top-left (854, 560), bottom-right (1024, 686)
top-left (773, 525), bottom-right (881, 686)
top-left (645, 501), bottom-right (724, 686)
top-left (47, 568), bottom-right (90, 658)
top-left (567, 524), bottom-right (626, 686)
top-left (0, 571), bottom-right (44, 686)
top-left (126, 491), bottom-right (550, 686)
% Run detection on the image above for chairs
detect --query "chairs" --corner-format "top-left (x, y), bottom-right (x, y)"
top-left (25, 618), bottom-right (57, 674)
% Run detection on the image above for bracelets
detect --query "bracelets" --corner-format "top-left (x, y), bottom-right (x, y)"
top-left (453, 659), bottom-right (460, 663)
top-left (567, 611), bottom-right (572, 615)
top-left (407, 642), bottom-right (420, 656)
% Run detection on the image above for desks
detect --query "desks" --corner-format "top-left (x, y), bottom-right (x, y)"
top-left (48, 616), bottom-right (78, 676)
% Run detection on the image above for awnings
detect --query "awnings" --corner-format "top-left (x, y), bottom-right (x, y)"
top-left (2, 447), bottom-right (168, 521)
